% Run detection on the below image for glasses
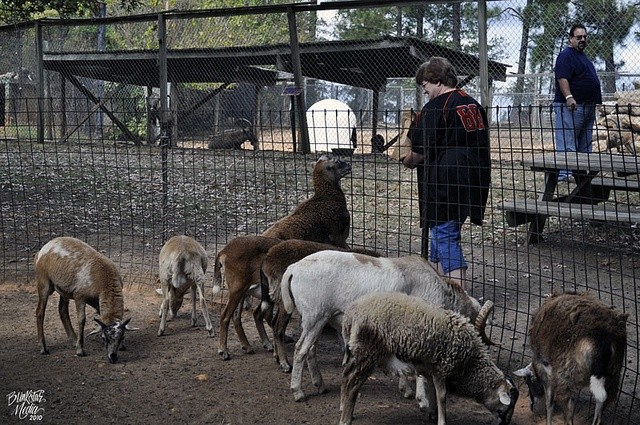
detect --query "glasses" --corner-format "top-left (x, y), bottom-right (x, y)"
top-left (573, 35), bottom-right (583, 40)
top-left (422, 80), bottom-right (429, 89)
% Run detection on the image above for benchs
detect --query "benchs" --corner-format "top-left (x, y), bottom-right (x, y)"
top-left (499, 196), bottom-right (637, 248)
top-left (564, 179), bottom-right (637, 230)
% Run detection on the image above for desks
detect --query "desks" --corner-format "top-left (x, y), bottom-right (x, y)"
top-left (525, 147), bottom-right (635, 255)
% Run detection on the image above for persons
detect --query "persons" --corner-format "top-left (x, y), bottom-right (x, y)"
top-left (550, 21), bottom-right (603, 183)
top-left (401, 55), bottom-right (491, 292)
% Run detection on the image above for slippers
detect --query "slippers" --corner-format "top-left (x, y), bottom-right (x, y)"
top-left (474, 299), bottom-right (494, 332)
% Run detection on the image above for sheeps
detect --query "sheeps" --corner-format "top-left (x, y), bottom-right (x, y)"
top-left (155, 235), bottom-right (214, 335)
top-left (260, 239), bottom-right (383, 371)
top-left (340, 292), bottom-right (519, 423)
top-left (212, 235), bottom-right (285, 359)
top-left (253, 154), bottom-right (353, 350)
top-left (513, 294), bottom-right (627, 424)
top-left (208, 117), bottom-right (260, 149)
top-left (280, 249), bottom-right (495, 402)
top-left (35, 235), bottom-right (139, 363)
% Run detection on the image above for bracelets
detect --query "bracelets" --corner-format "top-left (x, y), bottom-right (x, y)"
top-left (565, 93), bottom-right (573, 101)
